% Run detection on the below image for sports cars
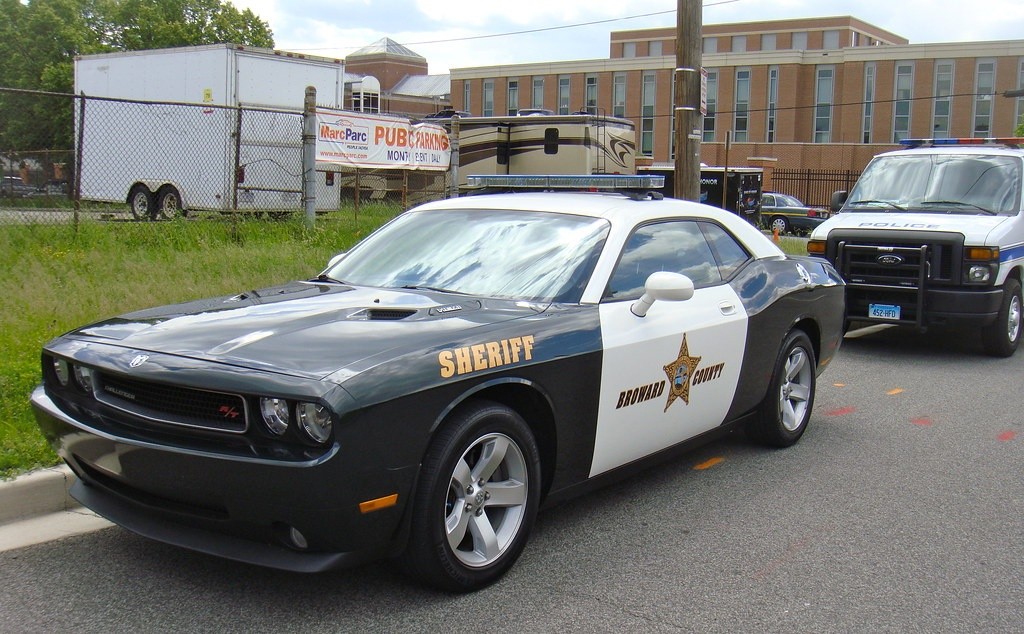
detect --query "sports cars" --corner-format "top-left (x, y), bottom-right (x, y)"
top-left (29, 176), bottom-right (847, 595)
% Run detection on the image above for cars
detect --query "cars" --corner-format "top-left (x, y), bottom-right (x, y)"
top-left (761, 191), bottom-right (830, 234)
top-left (0, 176), bottom-right (29, 198)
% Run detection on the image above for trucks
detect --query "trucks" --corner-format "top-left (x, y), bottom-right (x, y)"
top-left (340, 104), bottom-right (637, 206)
top-left (635, 162), bottom-right (762, 233)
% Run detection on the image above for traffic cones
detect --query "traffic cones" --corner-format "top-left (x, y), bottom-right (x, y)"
top-left (772, 227), bottom-right (781, 242)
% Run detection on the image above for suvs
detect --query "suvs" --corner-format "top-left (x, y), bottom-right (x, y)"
top-left (807, 136), bottom-right (1024, 356)
